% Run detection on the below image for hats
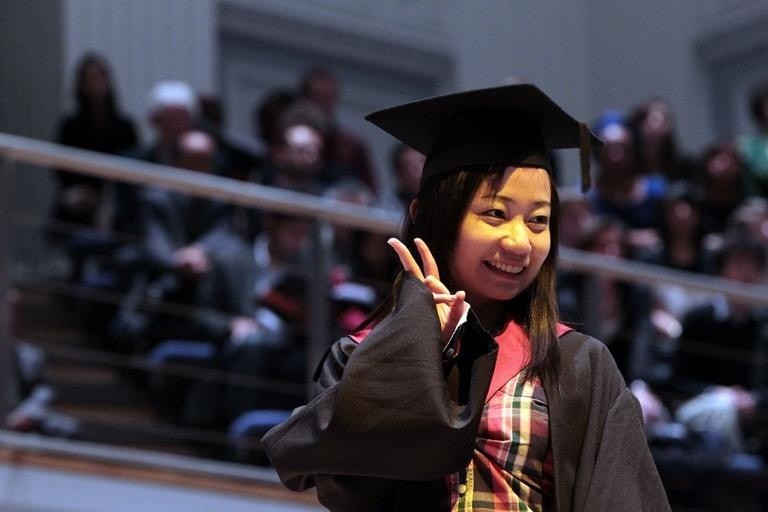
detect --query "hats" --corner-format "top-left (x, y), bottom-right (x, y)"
top-left (364, 81), bottom-right (606, 194)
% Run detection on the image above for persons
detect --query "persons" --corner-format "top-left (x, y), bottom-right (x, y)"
top-left (41, 52), bottom-right (427, 465)
top-left (261, 159), bottom-right (674, 511)
top-left (556, 96), bottom-right (768, 472)
